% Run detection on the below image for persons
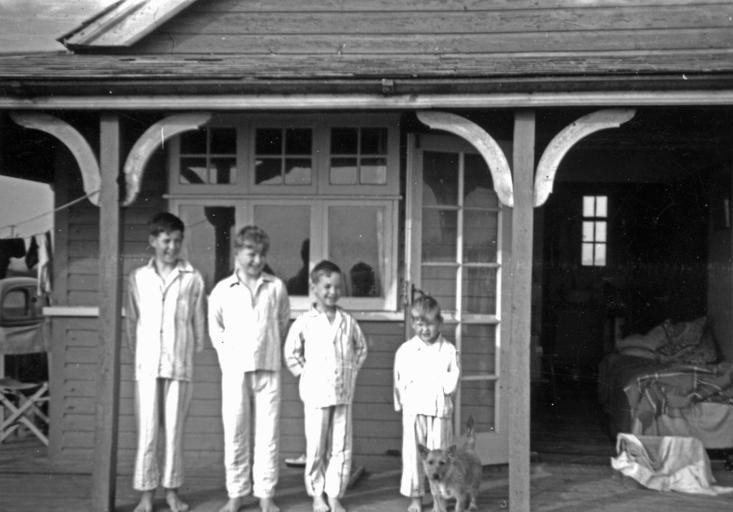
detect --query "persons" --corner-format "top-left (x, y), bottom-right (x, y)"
top-left (206, 225), bottom-right (290, 512)
top-left (286, 237), bottom-right (309, 295)
top-left (349, 261), bottom-right (375, 298)
top-left (393, 294), bottom-right (461, 512)
top-left (125, 211), bottom-right (206, 511)
top-left (282, 260), bottom-right (368, 512)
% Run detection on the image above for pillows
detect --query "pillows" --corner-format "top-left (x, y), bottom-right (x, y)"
top-left (615, 317), bottom-right (719, 364)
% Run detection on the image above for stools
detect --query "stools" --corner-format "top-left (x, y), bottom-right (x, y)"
top-left (0, 376), bottom-right (51, 448)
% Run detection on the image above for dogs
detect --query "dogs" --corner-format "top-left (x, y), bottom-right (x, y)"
top-left (416, 414), bottom-right (483, 512)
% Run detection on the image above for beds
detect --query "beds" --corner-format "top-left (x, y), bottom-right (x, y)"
top-left (596, 354), bottom-right (733, 470)
